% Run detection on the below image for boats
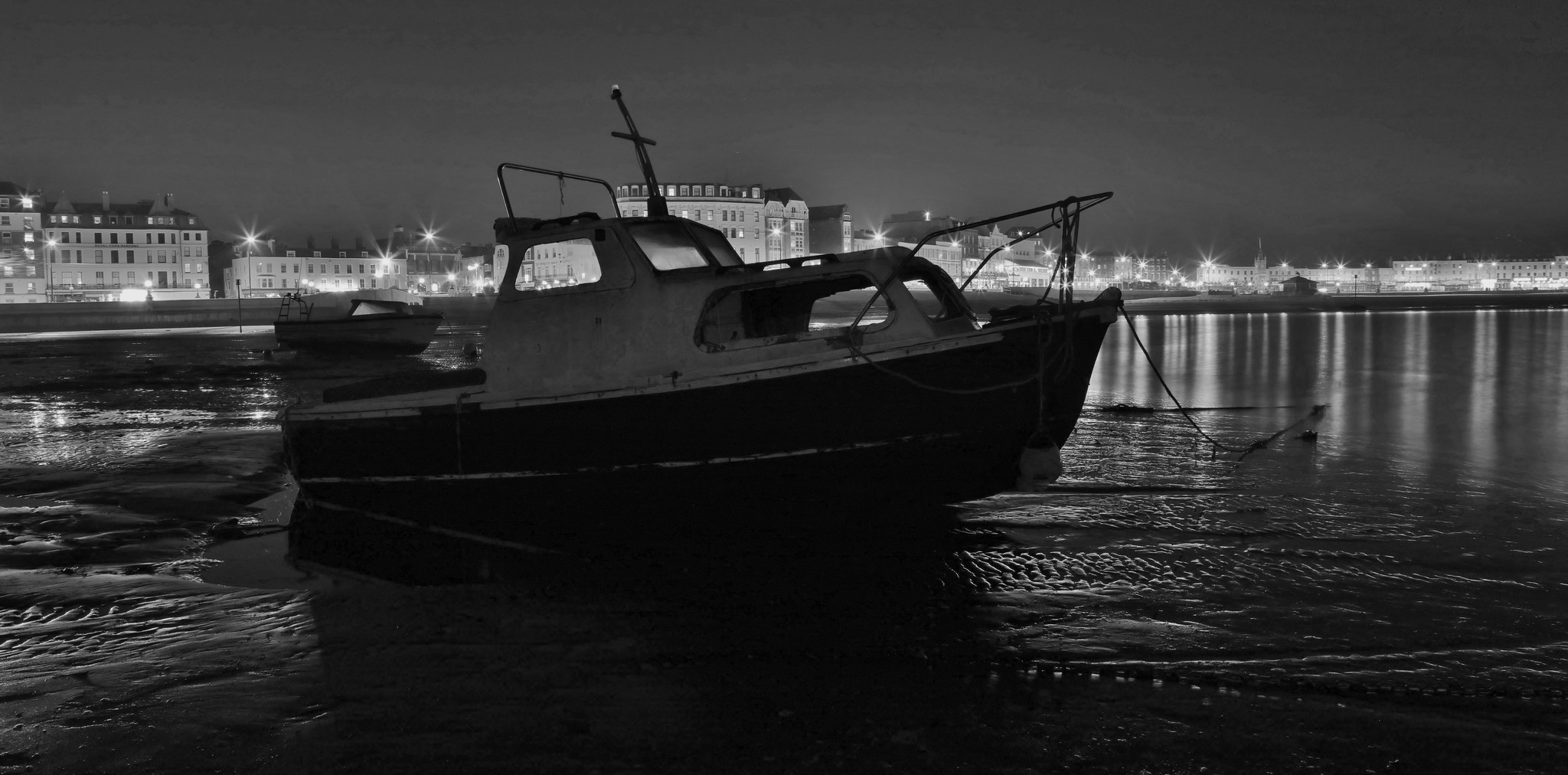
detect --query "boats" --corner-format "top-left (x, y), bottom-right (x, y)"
top-left (264, 82), bottom-right (1120, 526)
top-left (271, 255), bottom-right (447, 356)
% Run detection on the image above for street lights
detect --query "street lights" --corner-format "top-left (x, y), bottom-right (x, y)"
top-left (1206, 262), bottom-right (1210, 290)
top-left (1423, 264), bottom-right (1427, 289)
top-left (1492, 262), bottom-right (1496, 280)
top-left (953, 242), bottom-right (963, 288)
top-left (1478, 263), bottom-right (1482, 282)
top-left (245, 234), bottom-right (255, 296)
top-left (236, 279), bottom-right (242, 332)
top-left (423, 229), bottom-right (433, 296)
top-left (1366, 263), bottom-right (1372, 293)
top-left (475, 263), bottom-right (480, 294)
top-left (46, 238), bottom-right (56, 302)
top-left (1044, 249), bottom-right (1185, 290)
top-left (1354, 274), bottom-right (1358, 299)
top-left (1323, 264), bottom-right (1328, 291)
top-left (1283, 264), bottom-right (1288, 281)
top-left (1005, 246), bottom-right (1014, 288)
top-left (773, 227), bottom-right (783, 271)
top-left (1338, 265), bottom-right (1343, 287)
